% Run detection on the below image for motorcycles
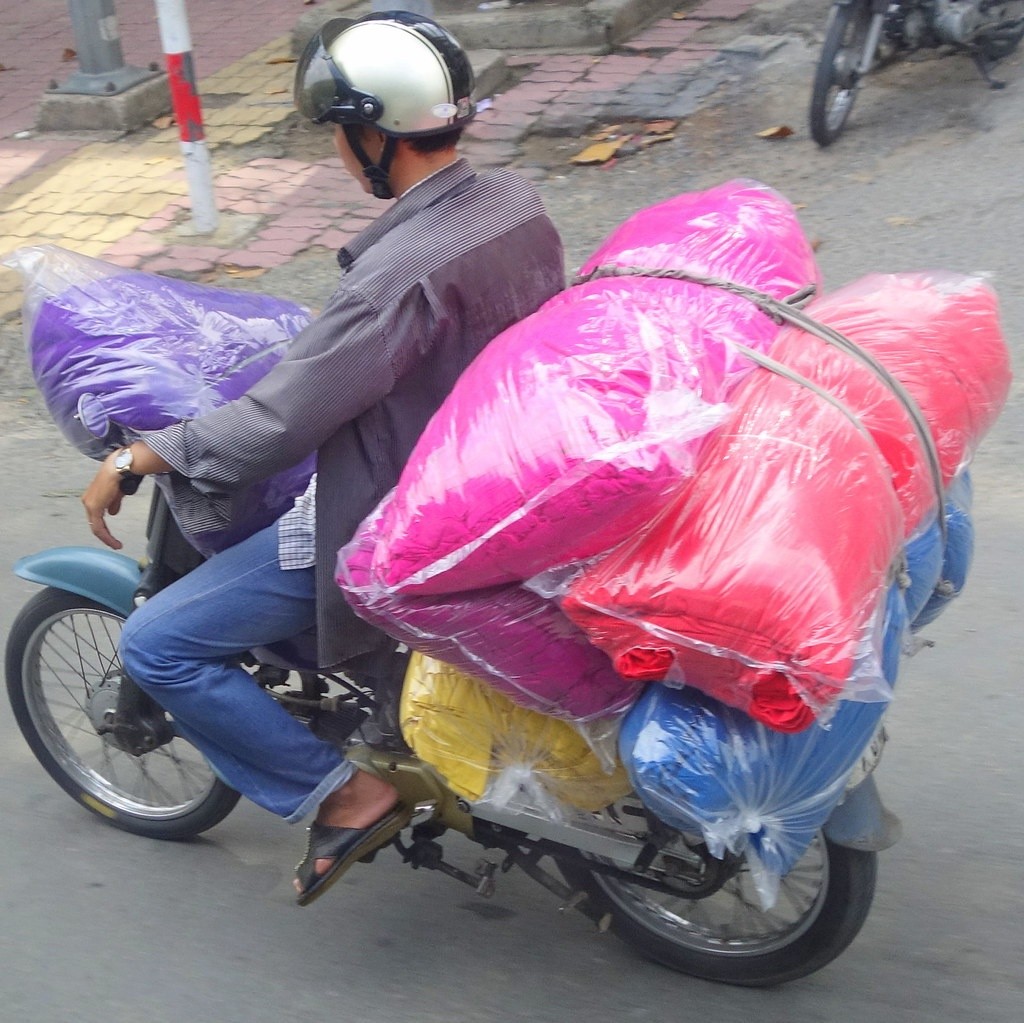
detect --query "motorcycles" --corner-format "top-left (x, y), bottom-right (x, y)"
top-left (806, 0), bottom-right (1024, 147)
top-left (3, 394), bottom-right (901, 990)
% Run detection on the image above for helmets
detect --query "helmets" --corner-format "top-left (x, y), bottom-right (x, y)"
top-left (311, 10), bottom-right (478, 140)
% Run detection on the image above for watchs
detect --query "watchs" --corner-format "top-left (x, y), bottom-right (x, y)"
top-left (115, 444), bottom-right (135, 478)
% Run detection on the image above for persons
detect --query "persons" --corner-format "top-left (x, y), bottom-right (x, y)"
top-left (83, 10), bottom-right (565, 906)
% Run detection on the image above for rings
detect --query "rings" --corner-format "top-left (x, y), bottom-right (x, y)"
top-left (87, 521), bottom-right (93, 525)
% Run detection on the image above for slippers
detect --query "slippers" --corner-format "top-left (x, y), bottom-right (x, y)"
top-left (294, 799), bottom-right (417, 908)
